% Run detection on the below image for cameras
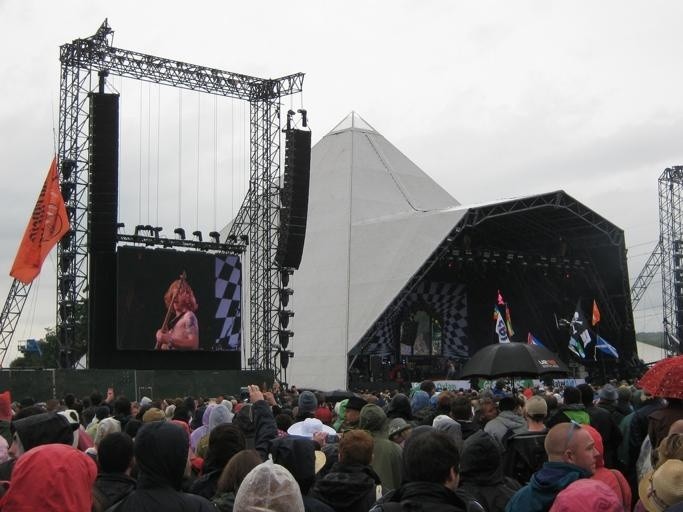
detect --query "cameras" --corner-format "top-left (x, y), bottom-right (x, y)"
top-left (240, 387), bottom-right (251, 400)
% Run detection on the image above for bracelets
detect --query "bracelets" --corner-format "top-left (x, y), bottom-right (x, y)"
top-left (167, 335), bottom-right (176, 350)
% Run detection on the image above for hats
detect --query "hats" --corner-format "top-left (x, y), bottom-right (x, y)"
top-left (525, 396), bottom-right (548, 416)
top-left (495, 381), bottom-right (505, 388)
top-left (638, 459), bottom-right (683, 511)
top-left (342, 397), bottom-right (368, 410)
top-left (668, 419), bottom-right (682, 437)
top-left (388, 417), bottom-right (412, 436)
top-left (599, 383), bottom-right (619, 401)
top-left (298, 390), bottom-right (317, 411)
top-left (287, 417), bottom-right (337, 438)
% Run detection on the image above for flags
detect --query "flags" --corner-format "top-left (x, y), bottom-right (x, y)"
top-left (560, 295), bottom-right (595, 364)
top-left (495, 313), bottom-right (512, 344)
top-left (528, 331), bottom-right (547, 350)
top-left (595, 333), bottom-right (619, 359)
top-left (496, 289), bottom-right (504, 307)
top-left (592, 295), bottom-right (602, 327)
top-left (504, 306), bottom-right (517, 336)
top-left (493, 302), bottom-right (502, 321)
top-left (6, 154), bottom-right (73, 287)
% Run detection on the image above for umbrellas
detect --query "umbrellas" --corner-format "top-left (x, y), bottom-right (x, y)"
top-left (455, 338), bottom-right (572, 399)
top-left (638, 355), bottom-right (683, 404)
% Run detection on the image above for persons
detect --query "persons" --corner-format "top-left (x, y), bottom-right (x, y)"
top-left (153, 276), bottom-right (201, 351)
top-left (1, 378), bottom-right (682, 511)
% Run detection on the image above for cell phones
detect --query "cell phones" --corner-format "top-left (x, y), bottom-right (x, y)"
top-left (325, 435), bottom-right (341, 444)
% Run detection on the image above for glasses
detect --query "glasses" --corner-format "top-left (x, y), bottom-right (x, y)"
top-left (563, 418), bottom-right (582, 452)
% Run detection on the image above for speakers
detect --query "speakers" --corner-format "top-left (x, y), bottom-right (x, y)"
top-left (274, 129), bottom-right (310, 269)
top-left (88, 92), bottom-right (120, 251)
